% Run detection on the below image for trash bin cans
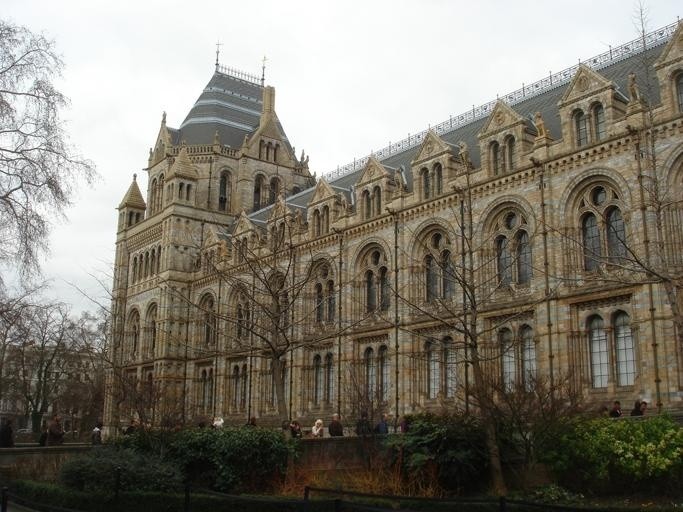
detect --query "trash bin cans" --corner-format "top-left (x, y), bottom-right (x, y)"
top-left (378, 423), bottom-right (387, 433)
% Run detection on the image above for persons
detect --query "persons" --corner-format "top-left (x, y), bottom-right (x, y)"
top-left (0, 412), bottom-right (64, 448)
top-left (356, 411), bottom-right (372, 435)
top-left (310, 419), bottom-right (323, 438)
top-left (329, 413), bottom-right (344, 436)
top-left (246, 417), bottom-right (257, 426)
top-left (282, 420), bottom-right (303, 439)
top-left (124, 420), bottom-right (137, 435)
top-left (211, 416), bottom-right (225, 429)
top-left (630, 401), bottom-right (647, 416)
top-left (609, 400), bottom-right (623, 417)
top-left (373, 413), bottom-right (388, 434)
top-left (91, 427), bottom-right (101, 444)
